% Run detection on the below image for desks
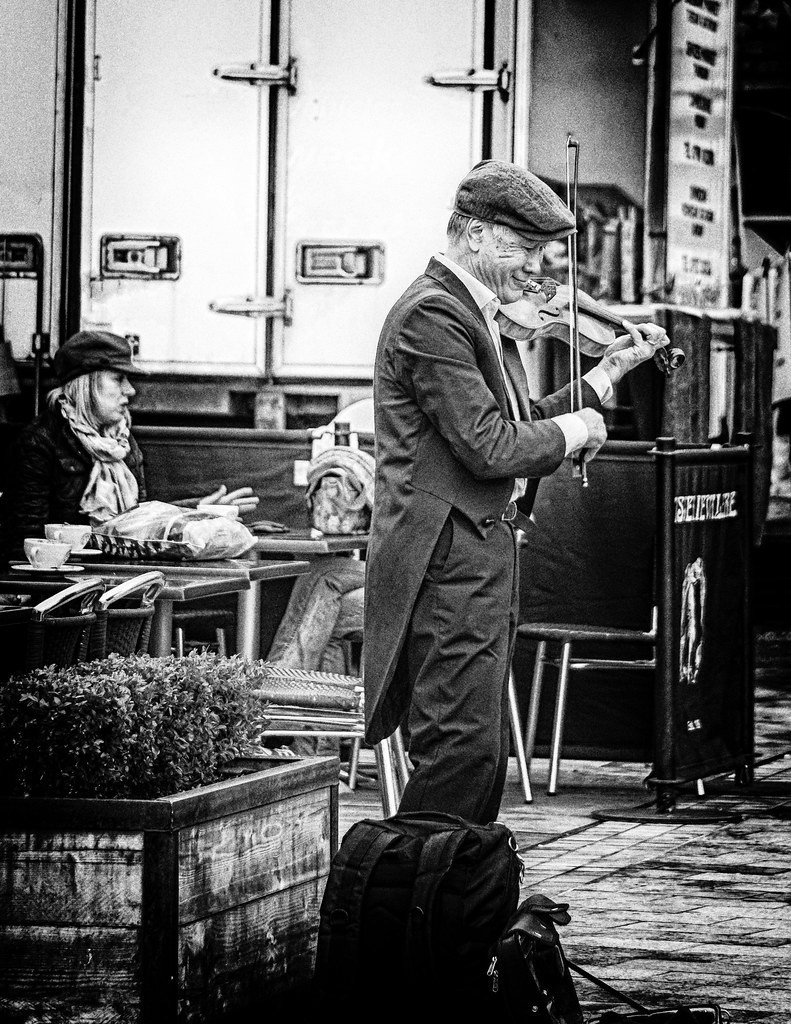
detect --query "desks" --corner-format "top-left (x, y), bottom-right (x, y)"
top-left (12, 559), bottom-right (311, 662)
top-left (1, 571), bottom-right (250, 659)
top-left (247, 532), bottom-right (371, 553)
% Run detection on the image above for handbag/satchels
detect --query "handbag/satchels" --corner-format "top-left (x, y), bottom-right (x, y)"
top-left (91, 501), bottom-right (256, 559)
top-left (309, 476), bottom-right (373, 534)
top-left (502, 896), bottom-right (585, 1024)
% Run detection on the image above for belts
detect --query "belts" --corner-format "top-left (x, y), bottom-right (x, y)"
top-left (501, 502), bottom-right (538, 534)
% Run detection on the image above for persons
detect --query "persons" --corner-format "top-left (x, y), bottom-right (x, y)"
top-left (256, 396), bottom-right (374, 759)
top-left (359, 155), bottom-right (669, 833)
top-left (0, 329), bottom-right (260, 564)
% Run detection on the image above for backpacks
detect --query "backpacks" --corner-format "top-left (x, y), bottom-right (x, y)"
top-left (315, 811), bottom-right (509, 1024)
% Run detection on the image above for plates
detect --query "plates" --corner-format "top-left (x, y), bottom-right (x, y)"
top-left (68, 546), bottom-right (105, 555)
top-left (14, 563), bottom-right (84, 574)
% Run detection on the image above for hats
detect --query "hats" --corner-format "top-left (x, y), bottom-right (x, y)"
top-left (53, 331), bottom-right (149, 384)
top-left (453, 159), bottom-right (578, 241)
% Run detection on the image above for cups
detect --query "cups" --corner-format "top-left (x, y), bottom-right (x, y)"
top-left (43, 524), bottom-right (90, 548)
top-left (22, 538), bottom-right (69, 565)
top-left (254, 392), bottom-right (288, 429)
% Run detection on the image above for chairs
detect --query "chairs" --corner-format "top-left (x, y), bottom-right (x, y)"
top-left (248, 620), bottom-right (402, 821)
top-left (517, 605), bottom-right (658, 797)
top-left (0, 577), bottom-right (106, 666)
top-left (88, 571), bottom-right (166, 661)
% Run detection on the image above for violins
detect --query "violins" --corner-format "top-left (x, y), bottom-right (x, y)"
top-left (493, 276), bottom-right (689, 371)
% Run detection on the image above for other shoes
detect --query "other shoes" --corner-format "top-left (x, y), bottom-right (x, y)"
top-left (261, 745), bottom-right (300, 758)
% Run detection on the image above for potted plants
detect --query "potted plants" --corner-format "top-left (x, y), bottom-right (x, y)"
top-left (0, 650), bottom-right (340, 1024)
